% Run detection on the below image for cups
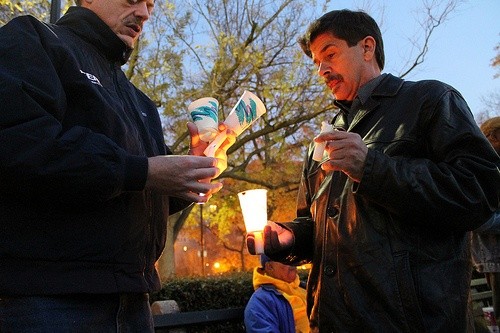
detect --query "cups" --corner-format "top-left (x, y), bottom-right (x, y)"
top-left (188, 97), bottom-right (219, 142)
top-left (482, 306), bottom-right (499, 333)
top-left (224, 90), bottom-right (266, 136)
top-left (237, 189), bottom-right (268, 234)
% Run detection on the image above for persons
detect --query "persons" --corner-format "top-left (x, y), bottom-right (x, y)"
top-left (482, 115), bottom-right (499, 152)
top-left (244, 257), bottom-right (314, 333)
top-left (244, 11), bottom-right (500, 333)
top-left (1, 0), bottom-right (219, 333)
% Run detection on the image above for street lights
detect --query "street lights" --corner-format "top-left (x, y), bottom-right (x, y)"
top-left (199, 192), bottom-right (211, 275)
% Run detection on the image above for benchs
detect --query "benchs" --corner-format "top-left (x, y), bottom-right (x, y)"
top-left (152, 308), bottom-right (244, 333)
top-left (470, 278), bottom-right (500, 333)
